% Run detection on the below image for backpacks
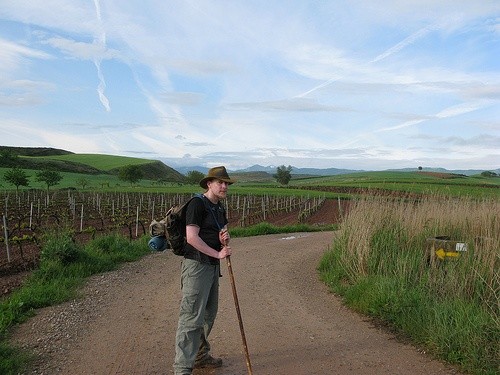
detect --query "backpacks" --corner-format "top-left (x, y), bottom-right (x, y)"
top-left (148, 195), bottom-right (206, 256)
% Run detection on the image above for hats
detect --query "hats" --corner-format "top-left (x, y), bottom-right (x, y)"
top-left (199, 166), bottom-right (237, 191)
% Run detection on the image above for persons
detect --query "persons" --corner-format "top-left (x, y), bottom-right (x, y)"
top-left (173, 166), bottom-right (235, 375)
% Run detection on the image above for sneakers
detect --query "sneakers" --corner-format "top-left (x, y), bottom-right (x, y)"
top-left (197, 355), bottom-right (222, 368)
